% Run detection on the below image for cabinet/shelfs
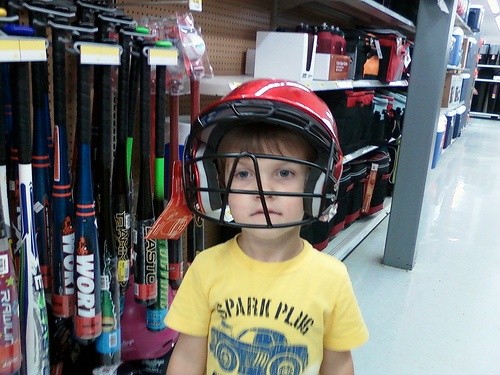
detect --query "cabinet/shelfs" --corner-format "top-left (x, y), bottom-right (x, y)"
top-left (164, 0), bottom-right (474, 261)
top-left (469, 64), bottom-right (500, 117)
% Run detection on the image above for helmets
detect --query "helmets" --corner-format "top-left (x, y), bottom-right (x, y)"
top-left (190, 79), bottom-right (343, 229)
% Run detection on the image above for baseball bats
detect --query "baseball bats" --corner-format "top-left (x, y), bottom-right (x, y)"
top-left (1, 0), bottom-right (200, 375)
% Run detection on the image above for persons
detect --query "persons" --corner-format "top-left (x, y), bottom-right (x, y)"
top-left (163, 78), bottom-right (369, 375)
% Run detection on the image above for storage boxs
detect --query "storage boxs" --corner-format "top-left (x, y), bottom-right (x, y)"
top-left (467, 5), bottom-right (484, 32)
top-left (443, 111), bottom-right (456, 149)
top-left (447, 26), bottom-right (464, 66)
top-left (244, 49), bottom-right (351, 80)
top-left (460, 73), bottom-right (470, 103)
top-left (457, 0), bottom-right (471, 23)
top-left (453, 105), bottom-right (466, 139)
top-left (441, 74), bottom-right (463, 108)
top-left (462, 38), bottom-right (469, 68)
top-left (431, 115), bottom-right (447, 169)
